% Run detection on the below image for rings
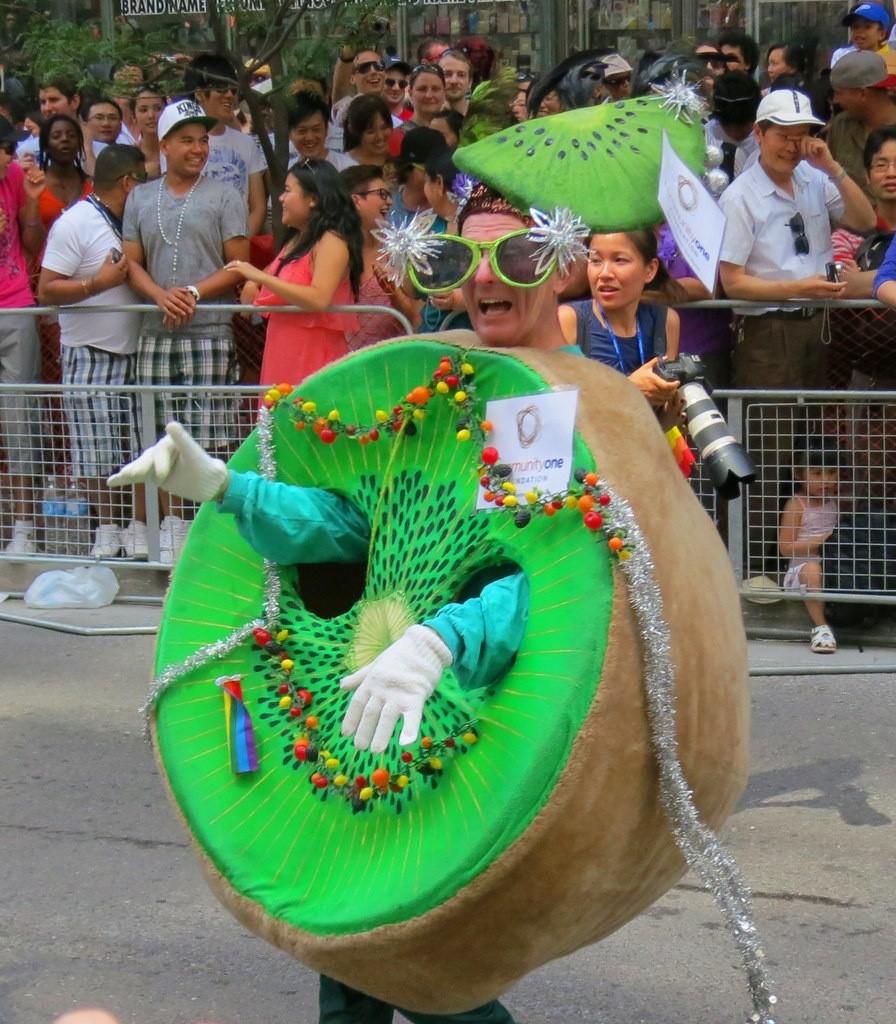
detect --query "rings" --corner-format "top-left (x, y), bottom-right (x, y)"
top-left (236, 259), bottom-right (240, 264)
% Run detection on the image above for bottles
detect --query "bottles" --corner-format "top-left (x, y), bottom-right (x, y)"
top-left (67, 476), bottom-right (91, 556)
top-left (43, 474), bottom-right (66, 554)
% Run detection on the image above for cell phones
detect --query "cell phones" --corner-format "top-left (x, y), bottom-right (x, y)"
top-left (110, 247), bottom-right (123, 263)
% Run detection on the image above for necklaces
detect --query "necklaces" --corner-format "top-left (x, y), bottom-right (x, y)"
top-left (156, 175), bottom-right (204, 286)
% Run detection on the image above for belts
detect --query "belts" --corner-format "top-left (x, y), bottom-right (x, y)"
top-left (764, 307), bottom-right (822, 320)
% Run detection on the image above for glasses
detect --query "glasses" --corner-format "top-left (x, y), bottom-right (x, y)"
top-left (299, 157), bottom-right (393, 200)
top-left (115, 171), bottom-right (148, 184)
top-left (249, 75), bottom-right (266, 83)
top-left (785, 211), bottom-right (809, 256)
top-left (870, 160), bottom-right (896, 172)
top-left (353, 60), bottom-right (410, 89)
top-left (407, 229), bottom-right (560, 294)
top-left (582, 70), bottom-right (631, 85)
top-left (412, 46), bottom-right (469, 76)
top-left (0, 141), bottom-right (18, 155)
top-left (698, 52), bottom-right (740, 68)
top-left (509, 70), bottom-right (557, 110)
top-left (137, 82), bottom-right (158, 93)
top-left (214, 80), bottom-right (240, 98)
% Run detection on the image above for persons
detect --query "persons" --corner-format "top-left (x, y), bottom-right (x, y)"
top-left (0, 3), bottom-right (895, 653)
top-left (106, 54), bottom-right (747, 1024)
top-left (122, 97), bottom-right (250, 565)
top-left (37, 144), bottom-right (149, 560)
top-left (0, 115), bottom-right (49, 565)
top-left (779, 439), bottom-right (845, 654)
top-left (719, 88), bottom-right (877, 607)
top-left (223, 156), bottom-right (365, 423)
top-left (558, 230), bottom-right (686, 436)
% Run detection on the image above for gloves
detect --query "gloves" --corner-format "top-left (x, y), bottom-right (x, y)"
top-left (107, 421), bottom-right (228, 502)
top-left (340, 624), bottom-right (453, 754)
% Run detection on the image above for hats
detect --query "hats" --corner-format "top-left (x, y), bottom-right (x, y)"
top-left (0, 115), bottom-right (30, 141)
top-left (590, 53), bottom-right (634, 79)
top-left (382, 56), bottom-right (412, 76)
top-left (710, 68), bottom-right (827, 131)
top-left (244, 58), bottom-right (271, 75)
top-left (829, 2), bottom-right (892, 93)
top-left (158, 99), bottom-right (219, 141)
top-left (385, 126), bottom-right (447, 163)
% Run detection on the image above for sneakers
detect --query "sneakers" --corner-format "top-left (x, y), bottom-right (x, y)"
top-left (2, 516), bottom-right (194, 564)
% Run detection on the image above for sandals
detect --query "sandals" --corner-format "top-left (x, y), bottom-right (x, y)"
top-left (809, 624), bottom-right (837, 653)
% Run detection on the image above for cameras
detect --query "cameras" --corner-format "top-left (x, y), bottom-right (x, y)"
top-left (651, 351), bottom-right (758, 499)
top-left (825, 262), bottom-right (839, 284)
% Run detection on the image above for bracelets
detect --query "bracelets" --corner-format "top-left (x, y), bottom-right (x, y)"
top-left (337, 47), bottom-right (357, 64)
top-left (91, 275), bottom-right (100, 295)
top-left (80, 279), bottom-right (94, 297)
top-left (826, 168), bottom-right (848, 184)
top-left (185, 285), bottom-right (202, 304)
top-left (23, 218), bottom-right (41, 227)
top-left (449, 291), bottom-right (456, 308)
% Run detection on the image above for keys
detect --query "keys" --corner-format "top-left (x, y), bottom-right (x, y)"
top-left (737, 321), bottom-right (744, 344)
top-left (742, 329), bottom-right (744, 342)
